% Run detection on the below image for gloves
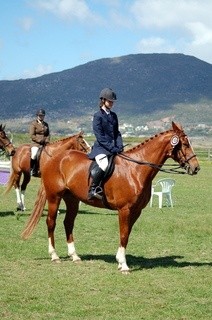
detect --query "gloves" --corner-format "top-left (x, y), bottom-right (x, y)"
top-left (111, 146), bottom-right (123, 154)
top-left (40, 140), bottom-right (48, 145)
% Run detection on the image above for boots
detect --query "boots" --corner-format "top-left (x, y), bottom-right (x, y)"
top-left (30, 158), bottom-right (38, 176)
top-left (88, 168), bottom-right (105, 200)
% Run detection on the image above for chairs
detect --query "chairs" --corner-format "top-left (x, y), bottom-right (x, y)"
top-left (150, 178), bottom-right (176, 208)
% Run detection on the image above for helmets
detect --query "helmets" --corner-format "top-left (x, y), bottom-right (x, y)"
top-left (36, 108), bottom-right (45, 116)
top-left (99, 88), bottom-right (118, 102)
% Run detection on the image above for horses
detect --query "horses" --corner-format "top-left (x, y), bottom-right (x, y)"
top-left (1, 123), bottom-right (17, 158)
top-left (20, 121), bottom-right (201, 275)
top-left (2, 130), bottom-right (92, 213)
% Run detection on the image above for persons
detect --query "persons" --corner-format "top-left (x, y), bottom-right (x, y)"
top-left (88, 87), bottom-right (124, 201)
top-left (30, 109), bottom-right (50, 177)
